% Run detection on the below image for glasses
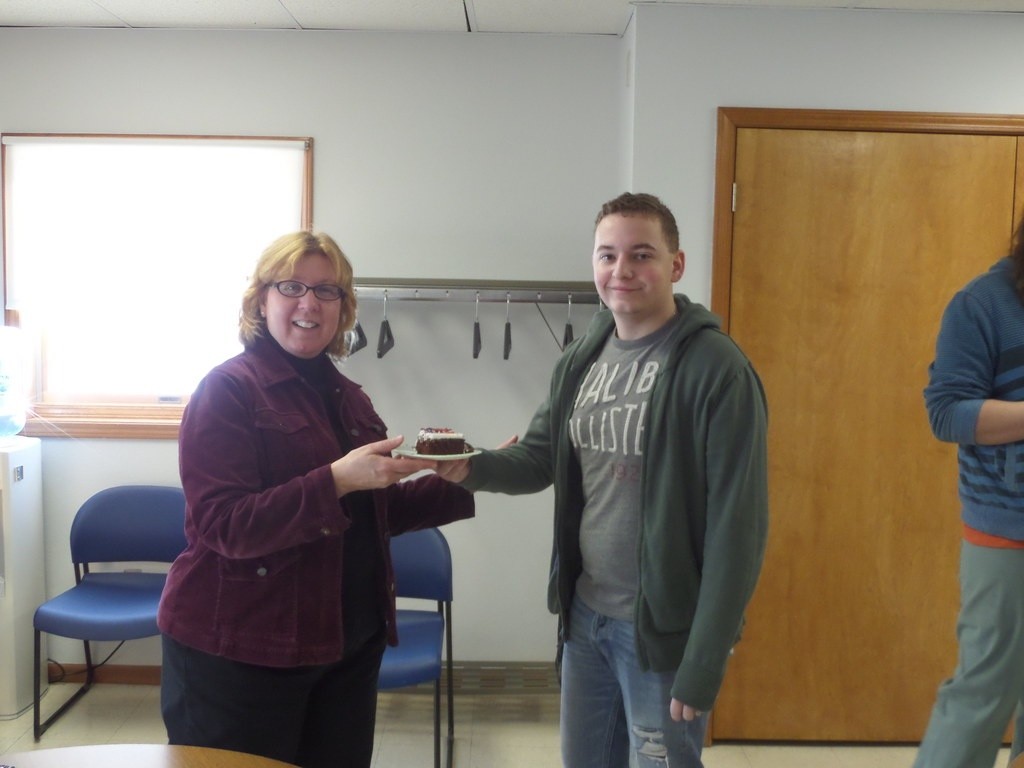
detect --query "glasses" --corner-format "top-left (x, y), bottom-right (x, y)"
top-left (275, 280), bottom-right (346, 301)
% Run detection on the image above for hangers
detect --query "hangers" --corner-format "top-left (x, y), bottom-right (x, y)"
top-left (342, 284), bottom-right (368, 358)
top-left (503, 293), bottom-right (512, 360)
top-left (472, 289), bottom-right (483, 359)
top-left (562, 295), bottom-right (573, 351)
top-left (378, 289), bottom-right (393, 358)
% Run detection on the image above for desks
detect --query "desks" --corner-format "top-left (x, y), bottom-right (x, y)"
top-left (0, 745), bottom-right (304, 768)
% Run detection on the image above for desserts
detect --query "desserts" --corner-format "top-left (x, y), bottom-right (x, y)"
top-left (415, 427), bottom-right (465, 454)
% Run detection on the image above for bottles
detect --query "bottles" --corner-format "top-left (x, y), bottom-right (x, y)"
top-left (0, 325), bottom-right (26, 434)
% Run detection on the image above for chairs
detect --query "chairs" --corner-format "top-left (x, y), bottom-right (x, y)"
top-left (30, 486), bottom-right (191, 745)
top-left (368, 521), bottom-right (453, 766)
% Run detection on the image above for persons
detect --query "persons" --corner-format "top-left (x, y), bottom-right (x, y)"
top-left (159, 231), bottom-right (518, 768)
top-left (434, 193), bottom-right (769, 768)
top-left (910, 204), bottom-right (1024, 768)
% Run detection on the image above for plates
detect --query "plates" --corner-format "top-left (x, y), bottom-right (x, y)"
top-left (393, 446), bottom-right (481, 460)
